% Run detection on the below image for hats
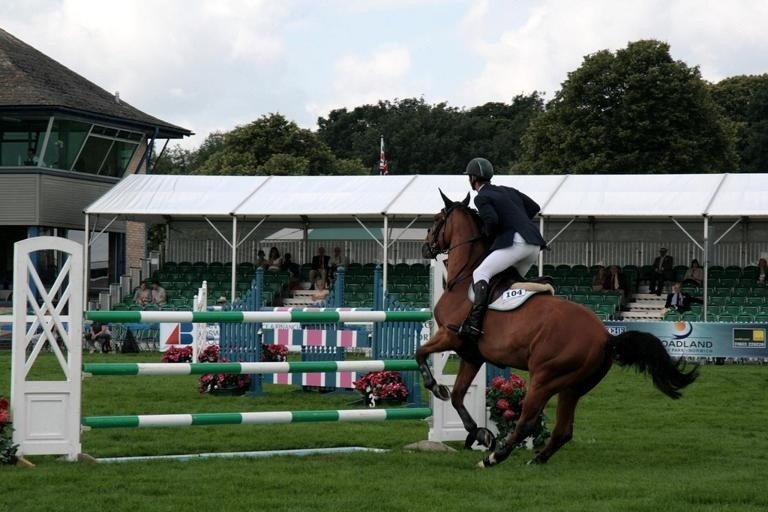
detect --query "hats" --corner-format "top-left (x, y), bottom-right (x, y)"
top-left (659, 248), bottom-right (670, 252)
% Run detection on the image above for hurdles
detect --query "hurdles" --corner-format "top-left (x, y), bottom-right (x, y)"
top-left (10, 236), bottom-right (487, 462)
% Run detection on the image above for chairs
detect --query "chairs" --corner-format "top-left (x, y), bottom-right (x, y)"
top-left (115, 260), bottom-right (654, 310)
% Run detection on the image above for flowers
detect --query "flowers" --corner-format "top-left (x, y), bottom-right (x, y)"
top-left (0, 395), bottom-right (20, 464)
top-left (262, 340), bottom-right (290, 361)
top-left (161, 343), bottom-right (193, 366)
top-left (352, 370), bottom-right (409, 408)
top-left (196, 345), bottom-right (251, 396)
top-left (484, 372), bottom-right (549, 447)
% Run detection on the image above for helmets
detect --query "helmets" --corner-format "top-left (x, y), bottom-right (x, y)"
top-left (463, 158), bottom-right (493, 180)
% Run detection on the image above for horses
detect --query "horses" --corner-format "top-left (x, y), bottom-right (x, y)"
top-left (413, 185), bottom-right (701, 469)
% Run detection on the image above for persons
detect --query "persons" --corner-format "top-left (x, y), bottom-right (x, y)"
top-left (82, 297), bottom-right (115, 355)
top-left (6, 291), bottom-right (13, 301)
top-left (752, 257), bottom-right (768, 288)
top-left (592, 264), bottom-right (629, 295)
top-left (252, 246), bottom-right (354, 304)
top-left (25, 308), bottom-right (66, 353)
top-left (649, 246), bottom-right (703, 314)
top-left (445, 157), bottom-right (552, 342)
top-left (150, 280), bottom-right (167, 305)
top-left (132, 281), bottom-right (152, 306)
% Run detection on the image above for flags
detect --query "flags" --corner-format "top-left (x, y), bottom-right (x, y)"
top-left (377, 137), bottom-right (389, 175)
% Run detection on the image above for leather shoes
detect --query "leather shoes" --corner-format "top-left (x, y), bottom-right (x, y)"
top-left (649, 289), bottom-right (661, 296)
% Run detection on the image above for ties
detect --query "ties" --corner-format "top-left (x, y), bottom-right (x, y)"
top-left (320, 256), bottom-right (322, 267)
top-left (612, 274), bottom-right (615, 290)
top-left (677, 293), bottom-right (679, 305)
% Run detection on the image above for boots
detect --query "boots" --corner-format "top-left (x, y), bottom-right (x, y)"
top-left (447, 280), bottom-right (488, 335)
